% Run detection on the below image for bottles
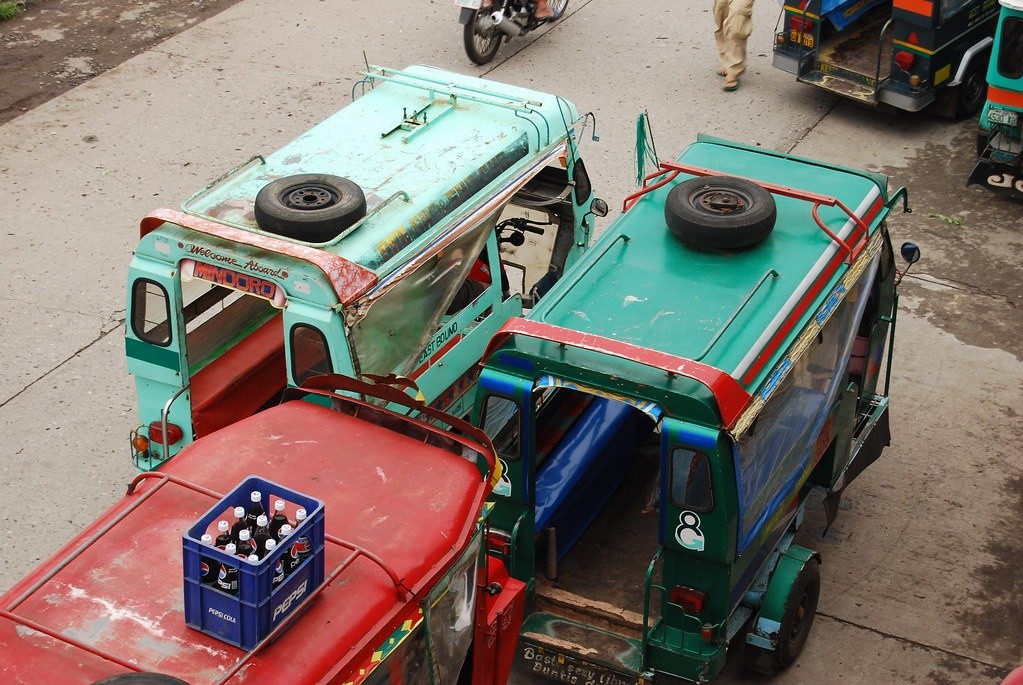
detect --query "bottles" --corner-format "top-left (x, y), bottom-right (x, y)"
top-left (201, 490), bottom-right (313, 595)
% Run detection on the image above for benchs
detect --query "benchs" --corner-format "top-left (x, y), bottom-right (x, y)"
top-left (186, 305), bottom-right (329, 438)
top-left (820, 0), bottom-right (881, 31)
top-left (742, 386), bottom-right (827, 508)
top-left (534, 388), bottom-right (654, 564)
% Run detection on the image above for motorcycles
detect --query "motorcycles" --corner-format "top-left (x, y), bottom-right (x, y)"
top-left (454, 0), bottom-right (572, 65)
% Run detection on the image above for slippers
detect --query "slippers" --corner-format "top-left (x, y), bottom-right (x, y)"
top-left (722, 78), bottom-right (739, 90)
top-left (537, 8), bottom-right (559, 20)
top-left (717, 69), bottom-right (726, 76)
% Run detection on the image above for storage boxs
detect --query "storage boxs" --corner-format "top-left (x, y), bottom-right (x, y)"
top-left (182, 475), bottom-right (326, 652)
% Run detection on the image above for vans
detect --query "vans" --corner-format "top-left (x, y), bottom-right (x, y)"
top-left (772, 1), bottom-right (1001, 123)
top-left (965, 0), bottom-right (1023, 201)
top-left (1, 49), bottom-right (919, 685)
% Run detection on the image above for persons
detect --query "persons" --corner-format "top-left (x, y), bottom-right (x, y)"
top-left (534, 0), bottom-right (559, 18)
top-left (405, 254), bottom-right (503, 310)
top-left (713, 0), bottom-right (756, 90)
